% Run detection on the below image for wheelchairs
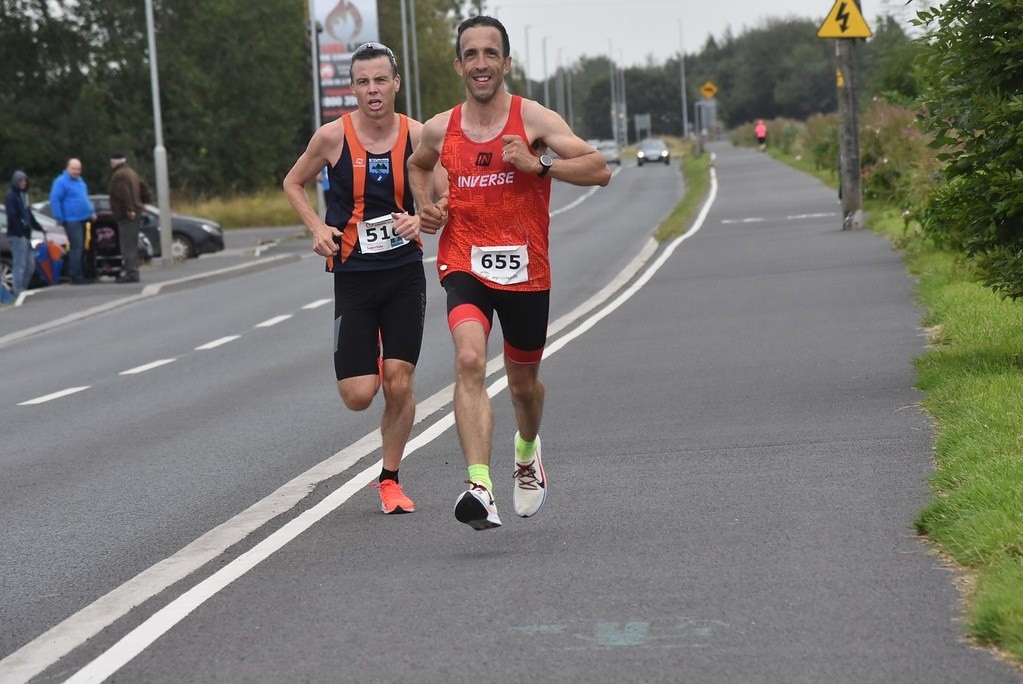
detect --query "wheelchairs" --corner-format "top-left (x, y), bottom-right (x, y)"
top-left (84, 210), bottom-right (128, 281)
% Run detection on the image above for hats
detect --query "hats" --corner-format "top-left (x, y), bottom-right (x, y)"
top-left (110, 150), bottom-right (124, 159)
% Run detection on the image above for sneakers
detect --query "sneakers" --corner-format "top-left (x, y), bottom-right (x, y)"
top-left (378, 329), bottom-right (383, 382)
top-left (368, 478), bottom-right (415, 514)
top-left (454, 480), bottom-right (502, 531)
top-left (513, 428), bottom-right (547, 517)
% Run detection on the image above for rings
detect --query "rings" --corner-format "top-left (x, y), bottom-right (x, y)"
top-left (503, 150), bottom-right (506, 156)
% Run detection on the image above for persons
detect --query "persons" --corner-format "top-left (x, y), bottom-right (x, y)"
top-left (282, 42), bottom-right (449, 516)
top-left (755, 118), bottom-right (767, 151)
top-left (407, 15), bottom-right (612, 531)
top-left (50, 158), bottom-right (100, 285)
top-left (109, 150), bottom-right (152, 283)
top-left (5, 172), bottom-right (48, 295)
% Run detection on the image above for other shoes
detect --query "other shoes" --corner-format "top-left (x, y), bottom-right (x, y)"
top-left (70, 275), bottom-right (95, 284)
top-left (117, 271), bottom-right (139, 283)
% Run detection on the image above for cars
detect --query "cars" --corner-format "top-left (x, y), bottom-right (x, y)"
top-left (30, 193), bottom-right (225, 267)
top-left (546, 136), bottom-right (622, 167)
top-left (635, 137), bottom-right (672, 166)
top-left (0, 202), bottom-right (72, 294)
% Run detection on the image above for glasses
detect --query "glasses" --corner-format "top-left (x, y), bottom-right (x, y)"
top-left (351, 42), bottom-right (397, 73)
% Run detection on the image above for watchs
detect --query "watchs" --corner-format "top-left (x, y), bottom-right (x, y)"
top-left (538, 155), bottom-right (552, 177)
